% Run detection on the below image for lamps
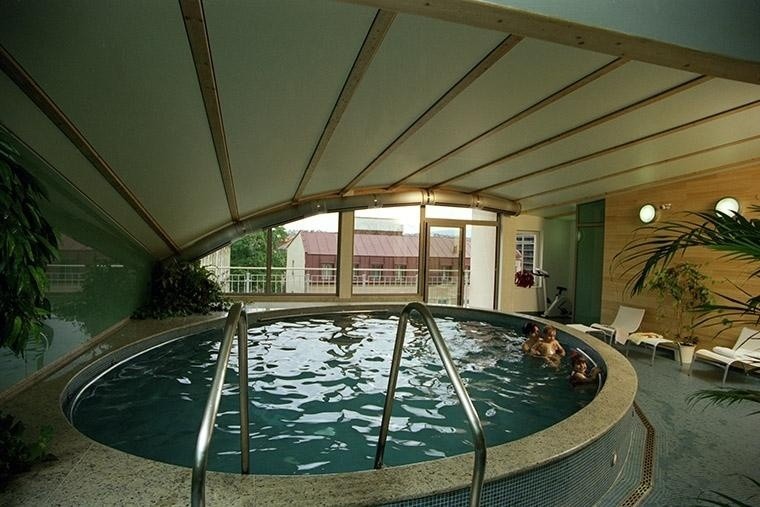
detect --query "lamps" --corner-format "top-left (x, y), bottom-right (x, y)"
top-left (638, 201), bottom-right (659, 223)
top-left (714, 195), bottom-right (741, 218)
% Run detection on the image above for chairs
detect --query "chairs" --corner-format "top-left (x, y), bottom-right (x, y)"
top-left (688, 326), bottom-right (759, 382)
top-left (567, 304), bottom-right (645, 347)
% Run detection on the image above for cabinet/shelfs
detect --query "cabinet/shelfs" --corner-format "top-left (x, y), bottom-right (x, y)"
top-left (516, 232), bottom-right (540, 285)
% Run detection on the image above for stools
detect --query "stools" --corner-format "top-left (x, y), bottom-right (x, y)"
top-left (625, 332), bottom-right (683, 366)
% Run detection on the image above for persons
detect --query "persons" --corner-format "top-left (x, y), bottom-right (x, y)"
top-left (567, 351), bottom-right (603, 387)
top-left (530, 325), bottom-right (566, 366)
top-left (520, 322), bottom-right (560, 356)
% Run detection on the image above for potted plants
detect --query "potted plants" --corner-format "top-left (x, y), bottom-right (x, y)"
top-left (642, 263), bottom-right (731, 363)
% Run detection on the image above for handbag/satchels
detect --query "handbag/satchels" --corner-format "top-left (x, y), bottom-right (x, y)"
top-left (517, 271), bottom-right (534, 287)
